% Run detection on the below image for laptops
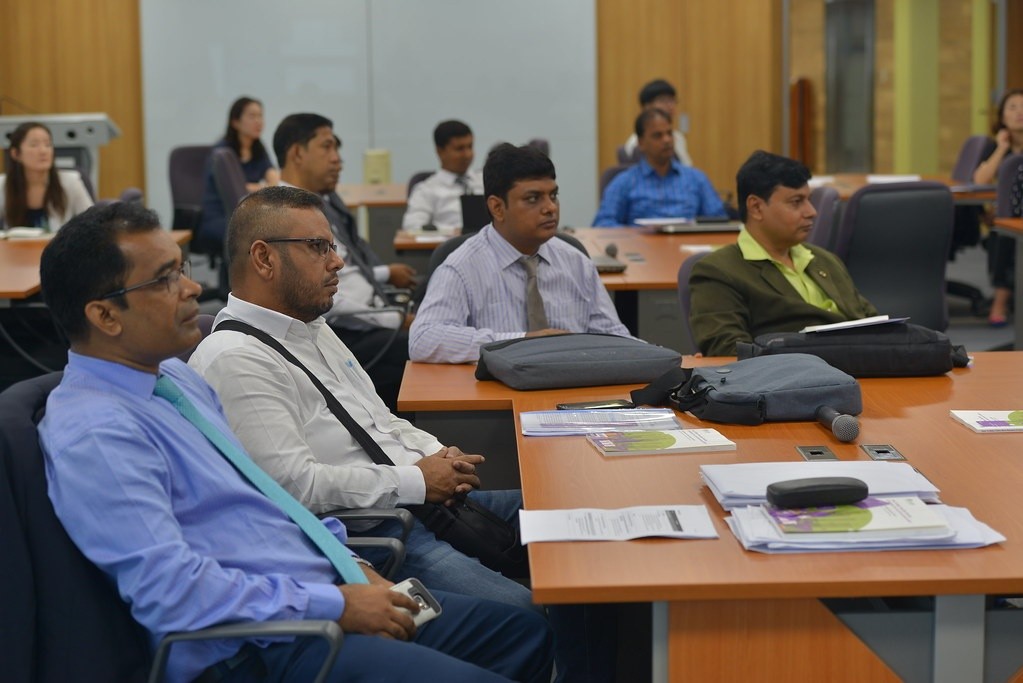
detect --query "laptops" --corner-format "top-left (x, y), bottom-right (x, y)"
top-left (591, 256), bottom-right (626, 273)
top-left (460, 194), bottom-right (495, 235)
top-left (658, 222), bottom-right (742, 234)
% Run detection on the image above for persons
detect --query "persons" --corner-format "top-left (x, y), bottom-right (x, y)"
top-left (187, 186), bottom-right (618, 683)
top-left (0, 123), bottom-right (95, 237)
top-left (190, 97), bottom-right (280, 264)
top-left (945, 89), bottom-right (1023, 328)
top-left (402, 121), bottom-right (484, 234)
top-left (409, 146), bottom-right (703, 365)
top-left (273, 112), bottom-right (422, 405)
top-left (625, 79), bottom-right (693, 166)
top-left (36, 202), bottom-right (555, 683)
top-left (689, 150), bottom-right (882, 356)
top-left (592, 108), bottom-right (730, 228)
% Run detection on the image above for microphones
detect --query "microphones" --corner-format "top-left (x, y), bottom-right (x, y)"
top-left (814, 405), bottom-right (860, 441)
top-left (605, 243), bottom-right (617, 257)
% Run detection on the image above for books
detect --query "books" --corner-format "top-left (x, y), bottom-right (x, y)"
top-left (586, 428), bottom-right (736, 457)
top-left (760, 494), bottom-right (946, 539)
top-left (798, 314), bottom-right (889, 333)
top-left (950, 410), bottom-right (1023, 434)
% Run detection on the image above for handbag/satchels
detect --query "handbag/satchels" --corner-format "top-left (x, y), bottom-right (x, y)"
top-left (631, 352), bottom-right (862, 424)
top-left (474, 333), bottom-right (683, 390)
top-left (407, 494), bottom-right (529, 579)
top-left (737, 321), bottom-right (969, 377)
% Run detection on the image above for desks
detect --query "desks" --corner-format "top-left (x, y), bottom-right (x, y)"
top-left (0, 182), bottom-right (1023, 683)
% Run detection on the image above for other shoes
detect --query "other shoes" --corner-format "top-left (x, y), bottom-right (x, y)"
top-left (989, 315), bottom-right (1007, 325)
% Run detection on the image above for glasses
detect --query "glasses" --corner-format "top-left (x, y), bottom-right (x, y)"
top-left (247, 238), bottom-right (339, 260)
top-left (104, 259), bottom-right (195, 300)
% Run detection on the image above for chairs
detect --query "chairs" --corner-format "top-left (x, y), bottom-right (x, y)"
top-left (598, 144), bottom-right (641, 194)
top-left (209, 146), bottom-right (259, 298)
top-left (806, 182), bottom-right (836, 251)
top-left (672, 251), bottom-right (714, 357)
top-left (993, 155), bottom-right (1023, 218)
top-left (0, 367), bottom-right (407, 683)
top-left (423, 228), bottom-right (588, 300)
top-left (951, 134), bottom-right (998, 180)
top-left (185, 312), bottom-right (414, 540)
top-left (166, 144), bottom-right (218, 271)
top-left (832, 179), bottom-right (961, 334)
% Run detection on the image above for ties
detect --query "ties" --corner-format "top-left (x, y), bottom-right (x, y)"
top-left (517, 254), bottom-right (548, 330)
top-left (153, 373), bottom-right (369, 586)
top-left (457, 177), bottom-right (474, 195)
